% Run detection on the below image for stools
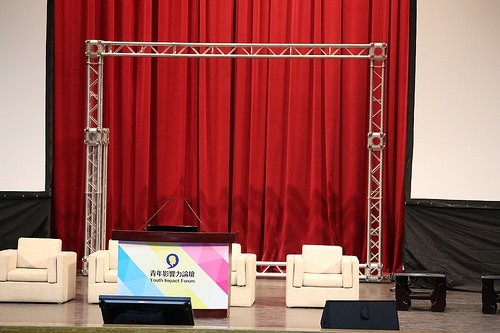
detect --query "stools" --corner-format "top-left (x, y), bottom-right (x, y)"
top-left (395, 273), bottom-right (447, 312)
top-left (481, 275), bottom-right (500, 315)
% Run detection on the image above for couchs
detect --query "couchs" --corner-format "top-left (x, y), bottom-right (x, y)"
top-left (88, 239), bottom-right (119, 304)
top-left (0, 237), bottom-right (77, 304)
top-left (286, 244), bottom-right (359, 308)
top-left (230, 242), bottom-right (257, 307)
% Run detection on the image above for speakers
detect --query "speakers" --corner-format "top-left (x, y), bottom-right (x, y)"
top-left (321, 300), bottom-right (399, 330)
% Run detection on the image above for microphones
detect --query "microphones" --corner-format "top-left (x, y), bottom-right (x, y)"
top-left (182, 198), bottom-right (206, 232)
top-left (141, 198), bottom-right (172, 231)
